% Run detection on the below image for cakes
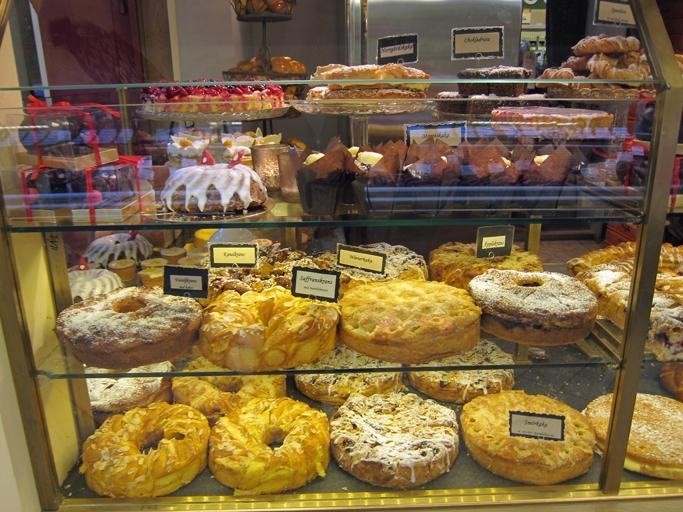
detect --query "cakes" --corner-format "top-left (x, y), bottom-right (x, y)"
top-left (166, 133), bottom-right (209, 158)
top-left (63, 270), bottom-right (122, 302)
top-left (221, 129), bottom-right (279, 159)
top-left (67, 233), bottom-right (155, 270)
top-left (144, 78), bottom-right (284, 112)
top-left (159, 163), bottom-right (267, 213)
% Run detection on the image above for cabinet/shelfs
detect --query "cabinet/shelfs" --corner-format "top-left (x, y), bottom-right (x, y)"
top-left (1, 1), bottom-right (683, 507)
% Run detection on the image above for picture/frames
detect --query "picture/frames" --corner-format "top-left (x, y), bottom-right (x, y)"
top-left (377, 32), bottom-right (418, 67)
top-left (451, 25), bottom-right (505, 60)
top-left (592, 0), bottom-right (640, 28)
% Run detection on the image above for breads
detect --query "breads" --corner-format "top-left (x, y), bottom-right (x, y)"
top-left (489, 65), bottom-right (532, 97)
top-left (138, 269), bottom-right (165, 287)
top-left (290, 136), bottom-right (346, 215)
top-left (161, 247), bottom-right (187, 264)
top-left (537, 66), bottom-right (590, 90)
top-left (659, 364), bottom-right (683, 399)
top-left (109, 260), bottom-right (135, 281)
top-left (228, 56), bottom-right (305, 73)
top-left (561, 53), bottom-right (590, 70)
top-left (330, 393), bottom-right (459, 488)
top-left (571, 32), bottom-right (641, 56)
top-left (428, 241), bottom-right (544, 288)
top-left (140, 258), bottom-right (168, 269)
top-left (409, 338), bottom-right (515, 404)
top-left (588, 50), bottom-right (650, 87)
top-left (466, 270), bottom-right (598, 346)
top-left (338, 280), bottom-right (480, 363)
top-left (306, 62), bottom-right (429, 90)
top-left (517, 140), bottom-right (574, 217)
top-left (343, 139), bottom-right (399, 216)
top-left (294, 343), bottom-right (403, 406)
top-left (313, 241), bottom-right (425, 289)
top-left (490, 106), bottom-right (614, 128)
top-left (55, 285), bottom-right (202, 370)
top-left (200, 237), bottom-right (313, 289)
top-left (209, 398), bottom-right (330, 494)
top-left (574, 241), bottom-right (683, 361)
top-left (436, 91), bottom-right (558, 113)
top-left (199, 291), bottom-right (340, 372)
top-left (581, 392), bottom-right (683, 481)
top-left (308, 86), bottom-right (428, 112)
top-left (458, 66), bottom-right (495, 96)
top-left (82, 402), bottom-right (210, 497)
top-left (461, 390), bottom-right (595, 485)
top-left (81, 361), bottom-right (176, 423)
top-left (172, 356), bottom-right (286, 412)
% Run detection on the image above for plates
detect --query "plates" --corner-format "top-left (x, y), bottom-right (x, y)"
top-left (136, 104), bottom-right (290, 120)
top-left (291, 99), bottom-right (431, 113)
top-left (433, 97), bottom-right (636, 112)
top-left (140, 195), bottom-right (276, 223)
